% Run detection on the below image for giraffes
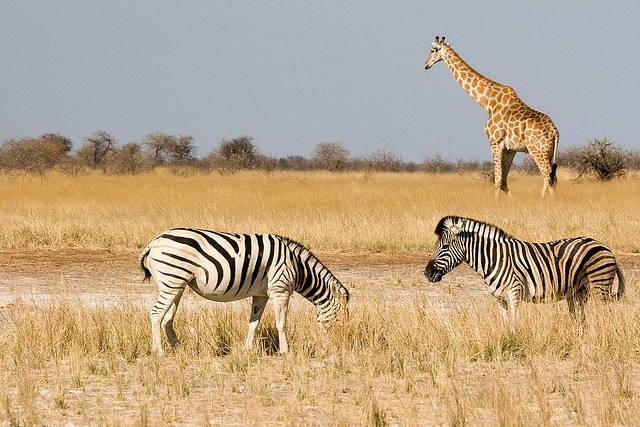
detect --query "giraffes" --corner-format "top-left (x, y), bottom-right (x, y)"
top-left (425, 35), bottom-right (559, 200)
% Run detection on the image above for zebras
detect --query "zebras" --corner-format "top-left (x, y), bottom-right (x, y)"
top-left (139, 227), bottom-right (350, 357)
top-left (424, 215), bottom-right (625, 323)
